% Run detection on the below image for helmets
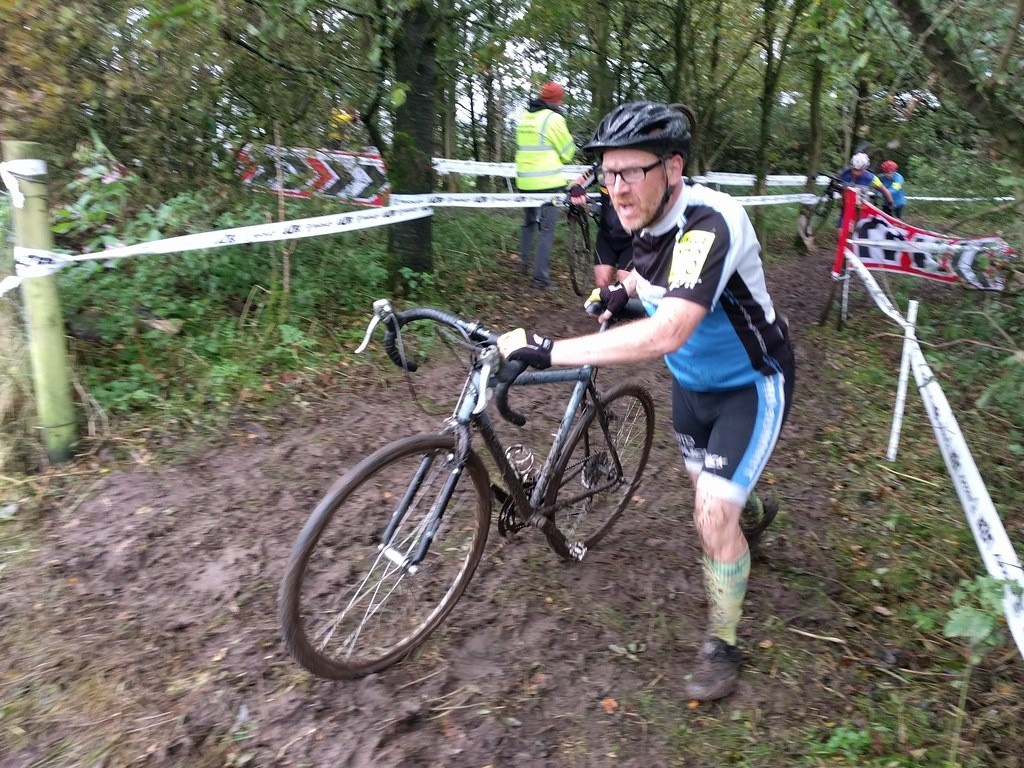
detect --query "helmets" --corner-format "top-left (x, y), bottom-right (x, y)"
top-left (850, 152), bottom-right (870, 170)
top-left (583, 100), bottom-right (693, 165)
top-left (881, 160), bottom-right (899, 173)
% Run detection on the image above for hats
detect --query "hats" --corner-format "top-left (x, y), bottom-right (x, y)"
top-left (541, 82), bottom-right (565, 103)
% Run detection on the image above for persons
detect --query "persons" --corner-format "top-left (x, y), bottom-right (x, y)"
top-left (327, 108), bottom-right (358, 150)
top-left (566, 159), bottom-right (644, 324)
top-left (494, 99), bottom-right (797, 703)
top-left (514, 81), bottom-right (577, 287)
top-left (873, 160), bottom-right (905, 220)
top-left (832, 152), bottom-right (892, 248)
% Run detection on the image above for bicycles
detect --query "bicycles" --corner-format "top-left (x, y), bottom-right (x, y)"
top-left (278, 282), bottom-right (657, 679)
top-left (563, 173), bottom-right (611, 300)
top-left (803, 170), bottom-right (852, 237)
top-left (867, 192), bottom-right (887, 211)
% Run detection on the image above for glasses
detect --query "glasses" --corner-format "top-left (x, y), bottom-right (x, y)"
top-left (593, 160), bottom-right (663, 186)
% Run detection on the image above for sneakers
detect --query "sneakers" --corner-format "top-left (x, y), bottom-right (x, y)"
top-left (739, 489), bottom-right (780, 540)
top-left (685, 635), bottom-right (743, 702)
